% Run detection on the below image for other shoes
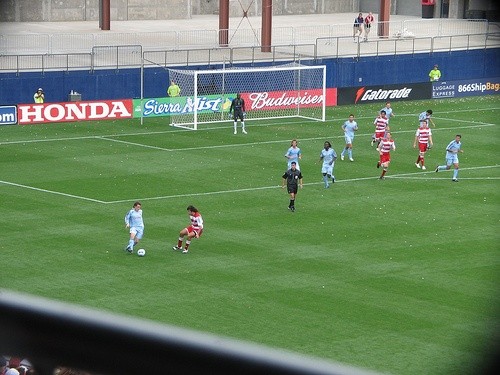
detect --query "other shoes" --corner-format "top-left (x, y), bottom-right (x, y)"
top-left (126, 245), bottom-right (131, 251)
top-left (128, 249), bottom-right (133, 254)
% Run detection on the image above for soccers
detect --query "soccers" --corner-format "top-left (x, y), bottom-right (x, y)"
top-left (138, 249), bottom-right (145, 256)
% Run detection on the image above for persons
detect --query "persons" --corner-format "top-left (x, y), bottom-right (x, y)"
top-left (167, 80), bottom-right (181, 97)
top-left (341, 114), bottom-right (359, 161)
top-left (229, 93), bottom-right (248, 134)
top-left (371, 101), bottom-right (396, 180)
top-left (353, 13), bottom-right (364, 43)
top-left (172, 205), bottom-right (203, 253)
top-left (363, 11), bottom-right (374, 40)
top-left (429, 65), bottom-right (442, 81)
top-left (125, 202), bottom-right (144, 254)
top-left (282, 140), bottom-right (303, 212)
top-left (0, 356), bottom-right (91, 375)
top-left (33, 88), bottom-right (44, 103)
top-left (318, 141), bottom-right (338, 189)
top-left (413, 109), bottom-right (435, 170)
top-left (436, 134), bottom-right (463, 182)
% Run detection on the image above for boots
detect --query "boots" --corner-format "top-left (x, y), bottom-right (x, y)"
top-left (241, 121), bottom-right (247, 134)
top-left (234, 121), bottom-right (238, 134)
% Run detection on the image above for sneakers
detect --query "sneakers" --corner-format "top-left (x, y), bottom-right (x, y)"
top-left (415, 162), bottom-right (421, 169)
top-left (377, 161), bottom-right (381, 168)
top-left (378, 177), bottom-right (385, 181)
top-left (421, 165), bottom-right (426, 170)
top-left (324, 183), bottom-right (330, 189)
top-left (371, 140), bottom-right (374, 147)
top-left (349, 158), bottom-right (354, 161)
top-left (288, 205), bottom-right (291, 210)
top-left (291, 205), bottom-right (296, 212)
top-left (452, 177), bottom-right (459, 182)
top-left (435, 165), bottom-right (440, 173)
top-left (332, 176), bottom-right (335, 183)
top-left (172, 245), bottom-right (182, 251)
top-left (341, 153), bottom-right (345, 160)
top-left (182, 249), bottom-right (189, 254)
